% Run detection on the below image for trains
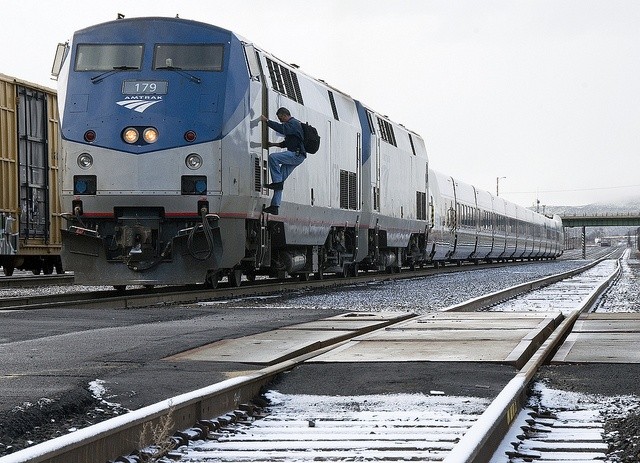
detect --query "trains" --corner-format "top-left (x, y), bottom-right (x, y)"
top-left (50, 14), bottom-right (564, 290)
top-left (601, 238), bottom-right (611, 247)
top-left (0, 72), bottom-right (66, 276)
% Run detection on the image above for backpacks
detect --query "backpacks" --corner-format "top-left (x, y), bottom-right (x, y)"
top-left (295, 121), bottom-right (320, 154)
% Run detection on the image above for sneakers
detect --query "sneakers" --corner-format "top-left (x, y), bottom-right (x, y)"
top-left (264, 206), bottom-right (278, 215)
top-left (265, 183), bottom-right (283, 190)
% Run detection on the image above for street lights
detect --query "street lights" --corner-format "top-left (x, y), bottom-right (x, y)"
top-left (497, 176), bottom-right (506, 196)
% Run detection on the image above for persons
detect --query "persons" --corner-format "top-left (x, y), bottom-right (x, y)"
top-left (259, 105), bottom-right (307, 215)
